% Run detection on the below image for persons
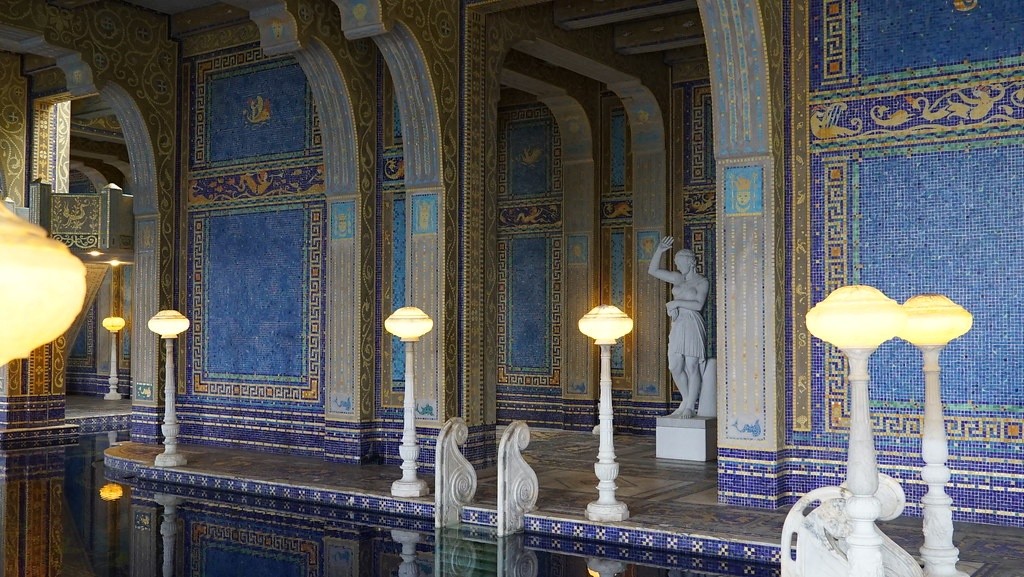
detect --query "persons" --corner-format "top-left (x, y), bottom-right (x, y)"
top-left (648, 236), bottom-right (709, 419)
